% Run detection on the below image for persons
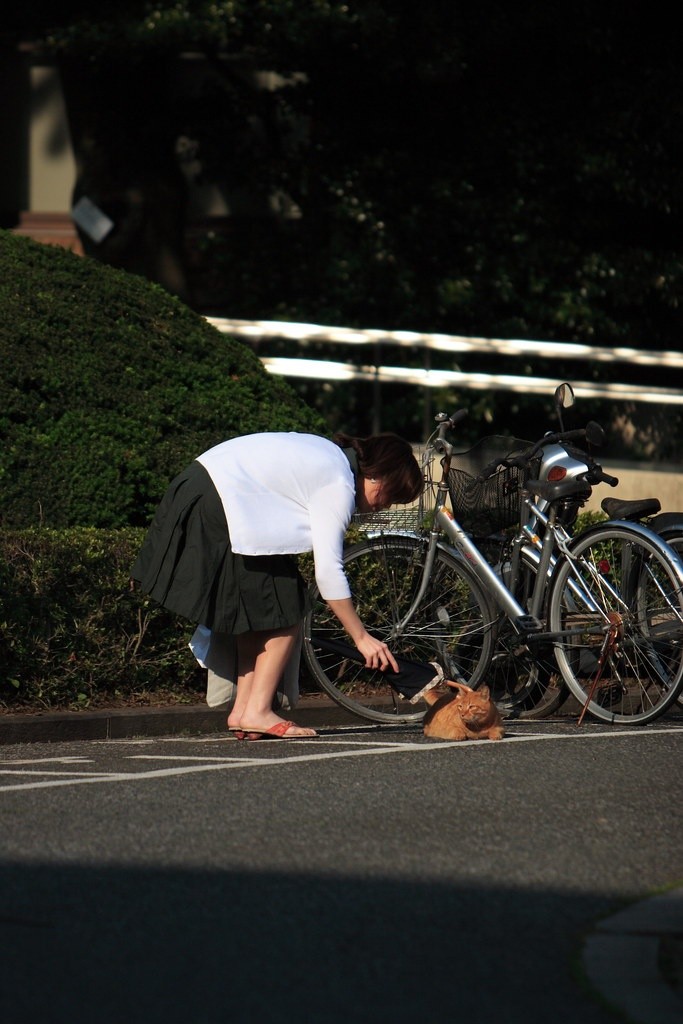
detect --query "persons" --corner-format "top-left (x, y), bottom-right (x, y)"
top-left (130, 432), bottom-right (423, 739)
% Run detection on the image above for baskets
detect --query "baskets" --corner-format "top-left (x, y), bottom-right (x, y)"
top-left (351, 446), bottom-right (433, 549)
top-left (440, 435), bottom-right (543, 533)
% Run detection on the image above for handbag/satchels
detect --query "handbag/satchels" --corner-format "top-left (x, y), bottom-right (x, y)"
top-left (187, 615), bottom-right (303, 711)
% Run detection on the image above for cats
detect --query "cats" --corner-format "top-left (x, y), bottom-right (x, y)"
top-left (422, 685), bottom-right (506, 741)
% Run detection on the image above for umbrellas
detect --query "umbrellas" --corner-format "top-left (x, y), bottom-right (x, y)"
top-left (304, 637), bottom-right (474, 704)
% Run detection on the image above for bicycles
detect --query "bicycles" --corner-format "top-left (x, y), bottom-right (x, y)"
top-left (301, 409), bottom-right (683, 726)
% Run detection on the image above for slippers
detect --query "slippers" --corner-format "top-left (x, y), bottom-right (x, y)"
top-left (242, 715), bottom-right (319, 740)
top-left (229, 723), bottom-right (247, 739)
